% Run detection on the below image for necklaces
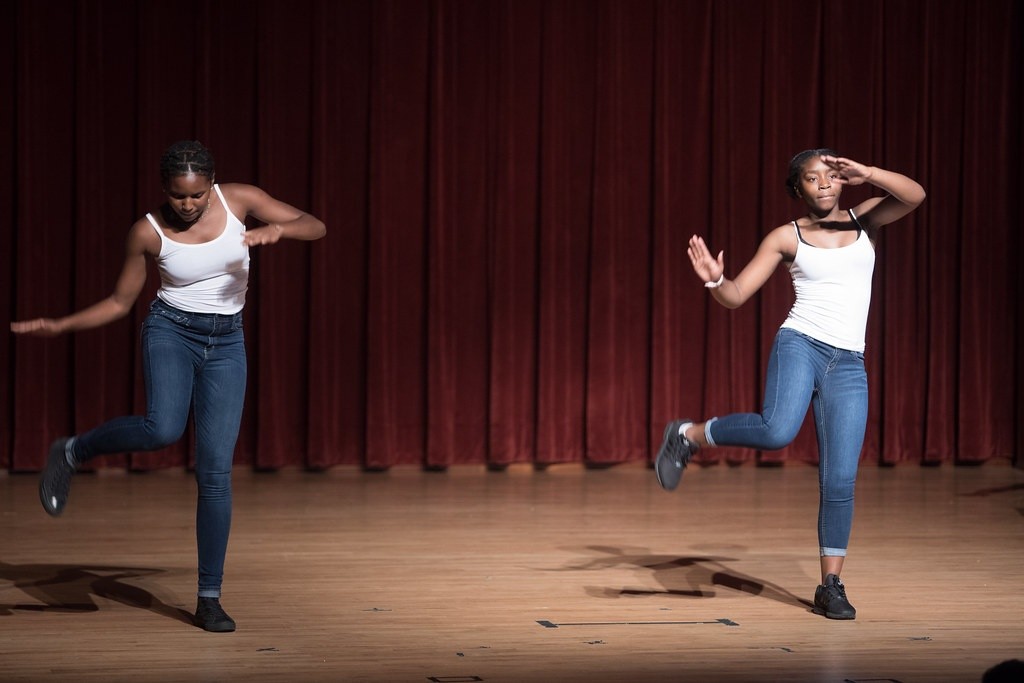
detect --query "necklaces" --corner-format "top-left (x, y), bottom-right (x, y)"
top-left (191, 197), bottom-right (210, 222)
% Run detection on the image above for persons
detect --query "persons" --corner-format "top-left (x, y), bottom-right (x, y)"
top-left (654, 149), bottom-right (925, 620)
top-left (10, 140), bottom-right (326, 632)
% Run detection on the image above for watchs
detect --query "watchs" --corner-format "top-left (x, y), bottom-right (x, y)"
top-left (704, 273), bottom-right (724, 288)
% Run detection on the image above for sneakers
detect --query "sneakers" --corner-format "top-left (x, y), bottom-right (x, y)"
top-left (655, 421), bottom-right (698, 490)
top-left (39, 438), bottom-right (73, 517)
top-left (813, 574), bottom-right (856, 619)
top-left (194, 596), bottom-right (236, 631)
